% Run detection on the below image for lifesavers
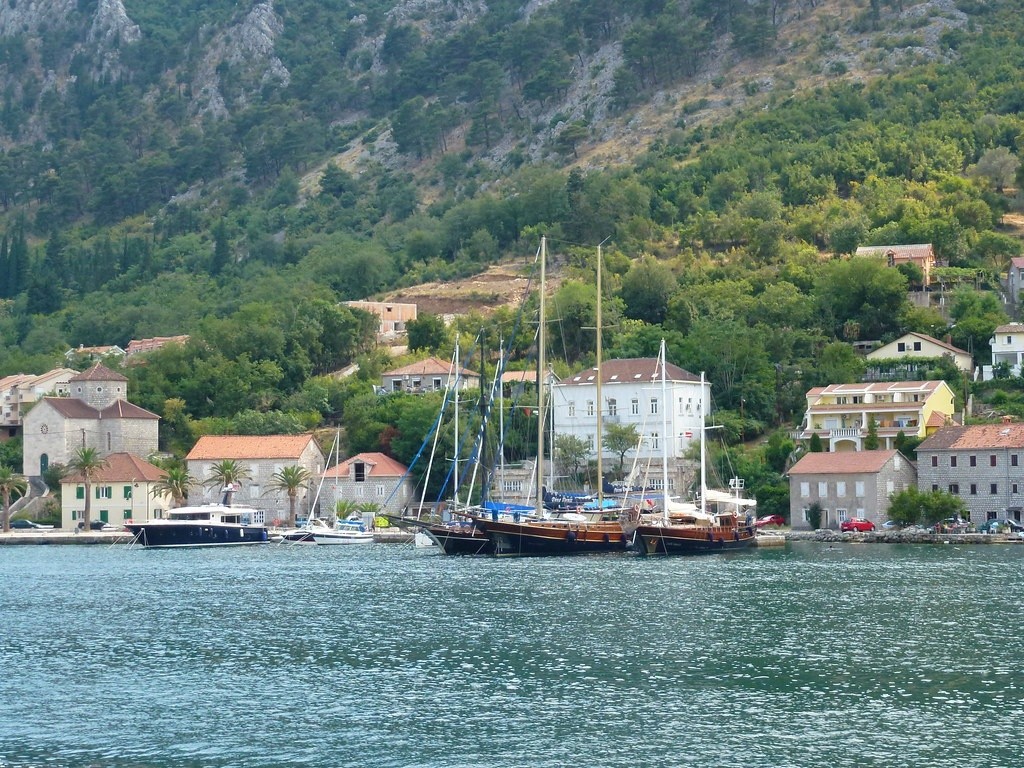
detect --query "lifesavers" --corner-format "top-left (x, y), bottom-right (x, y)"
top-left (505, 507), bottom-right (510, 514)
top-left (732, 511), bottom-right (739, 519)
top-left (576, 507), bottom-right (582, 514)
top-left (273, 518), bottom-right (280, 526)
top-left (634, 504), bottom-right (640, 512)
top-left (464, 525), bottom-right (471, 534)
top-left (453, 526), bottom-right (461, 534)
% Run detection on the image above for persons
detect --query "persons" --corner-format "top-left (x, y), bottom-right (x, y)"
top-left (936, 521), bottom-right (958, 534)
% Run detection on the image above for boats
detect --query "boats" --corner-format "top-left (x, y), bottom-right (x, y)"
top-left (271, 523), bottom-right (432, 547)
top-left (121, 502), bottom-right (270, 549)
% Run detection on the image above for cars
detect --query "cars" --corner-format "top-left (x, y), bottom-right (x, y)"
top-left (839, 517), bottom-right (876, 533)
top-left (340, 517), bottom-right (363, 524)
top-left (977, 518), bottom-right (1024, 534)
top-left (8, 519), bottom-right (38, 529)
top-left (78, 519), bottom-right (106, 531)
top-left (757, 515), bottom-right (784, 528)
top-left (295, 517), bottom-right (312, 527)
top-left (930, 519), bottom-right (967, 533)
top-left (882, 522), bottom-right (899, 528)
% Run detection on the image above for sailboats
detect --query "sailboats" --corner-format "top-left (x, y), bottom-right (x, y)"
top-left (379, 233), bottom-right (763, 557)
